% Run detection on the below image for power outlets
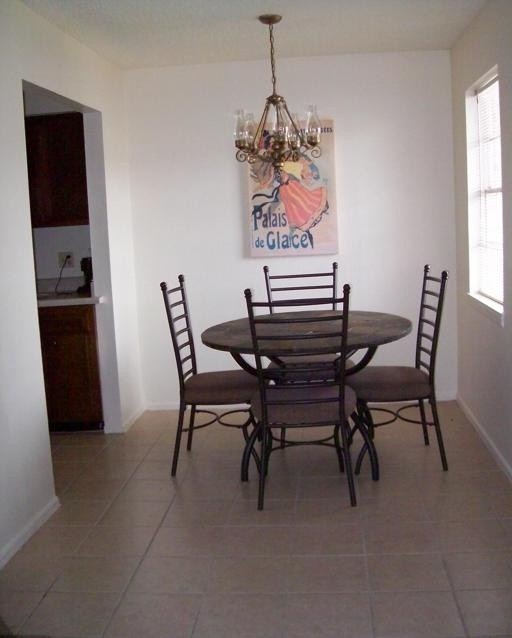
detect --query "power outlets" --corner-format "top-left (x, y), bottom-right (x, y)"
top-left (59, 249), bottom-right (74, 268)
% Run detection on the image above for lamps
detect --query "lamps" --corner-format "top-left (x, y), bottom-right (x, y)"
top-left (231, 13), bottom-right (324, 180)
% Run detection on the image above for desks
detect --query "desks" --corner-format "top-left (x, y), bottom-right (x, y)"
top-left (197, 308), bottom-right (416, 482)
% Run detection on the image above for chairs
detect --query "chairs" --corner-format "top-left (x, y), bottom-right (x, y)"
top-left (337, 260), bottom-right (450, 473)
top-left (158, 272), bottom-right (265, 477)
top-left (242, 284), bottom-right (358, 509)
top-left (259, 260), bottom-right (353, 451)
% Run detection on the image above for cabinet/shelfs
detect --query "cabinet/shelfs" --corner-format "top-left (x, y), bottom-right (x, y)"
top-left (24, 110), bottom-right (90, 228)
top-left (39, 308), bottom-right (104, 435)
